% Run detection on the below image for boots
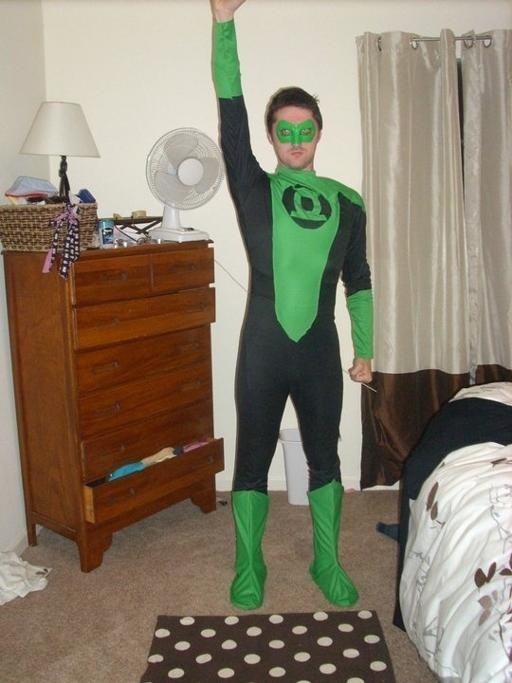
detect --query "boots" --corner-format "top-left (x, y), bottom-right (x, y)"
top-left (307, 480), bottom-right (358, 608)
top-left (229, 488), bottom-right (266, 610)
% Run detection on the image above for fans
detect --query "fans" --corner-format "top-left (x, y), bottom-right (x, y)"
top-left (146, 127), bottom-right (225, 242)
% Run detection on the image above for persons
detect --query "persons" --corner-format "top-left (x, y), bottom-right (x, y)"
top-left (210, 1), bottom-right (374, 610)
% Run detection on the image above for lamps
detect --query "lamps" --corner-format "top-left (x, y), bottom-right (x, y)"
top-left (20, 100), bottom-right (100, 198)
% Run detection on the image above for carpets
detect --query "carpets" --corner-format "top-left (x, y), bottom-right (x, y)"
top-left (139, 609), bottom-right (396, 683)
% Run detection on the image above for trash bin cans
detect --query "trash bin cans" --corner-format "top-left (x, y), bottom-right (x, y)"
top-left (278, 428), bottom-right (309, 505)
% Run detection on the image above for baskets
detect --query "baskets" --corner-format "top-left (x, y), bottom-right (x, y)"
top-left (0, 202), bottom-right (98, 253)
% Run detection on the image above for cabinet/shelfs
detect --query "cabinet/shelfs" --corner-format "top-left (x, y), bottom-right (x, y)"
top-left (0, 238), bottom-right (224, 574)
